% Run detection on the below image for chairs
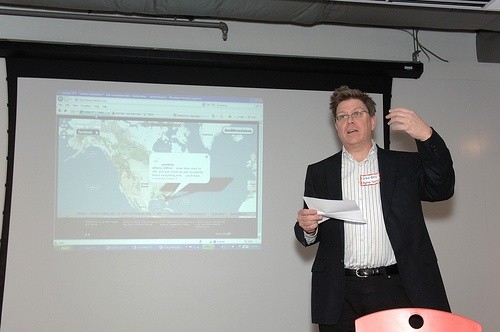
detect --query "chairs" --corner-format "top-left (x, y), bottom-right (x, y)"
top-left (355, 308), bottom-right (482, 332)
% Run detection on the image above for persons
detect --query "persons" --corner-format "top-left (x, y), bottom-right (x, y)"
top-left (294, 85), bottom-right (455, 332)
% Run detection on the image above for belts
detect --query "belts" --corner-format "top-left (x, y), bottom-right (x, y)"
top-left (344, 263), bottom-right (398, 278)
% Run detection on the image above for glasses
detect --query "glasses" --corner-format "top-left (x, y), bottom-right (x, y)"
top-left (333, 110), bottom-right (371, 120)
